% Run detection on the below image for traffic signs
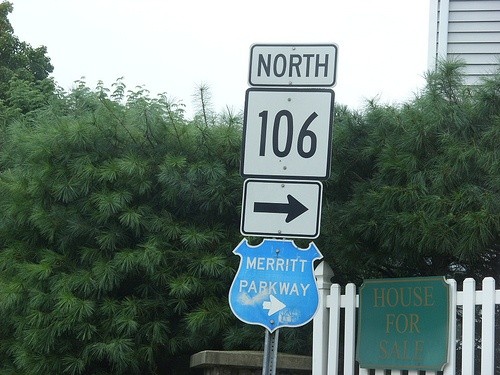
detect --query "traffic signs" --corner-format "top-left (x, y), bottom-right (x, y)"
top-left (228, 238), bottom-right (323, 333)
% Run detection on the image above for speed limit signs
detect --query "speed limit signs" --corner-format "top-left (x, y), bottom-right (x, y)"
top-left (242, 87), bottom-right (335, 180)
